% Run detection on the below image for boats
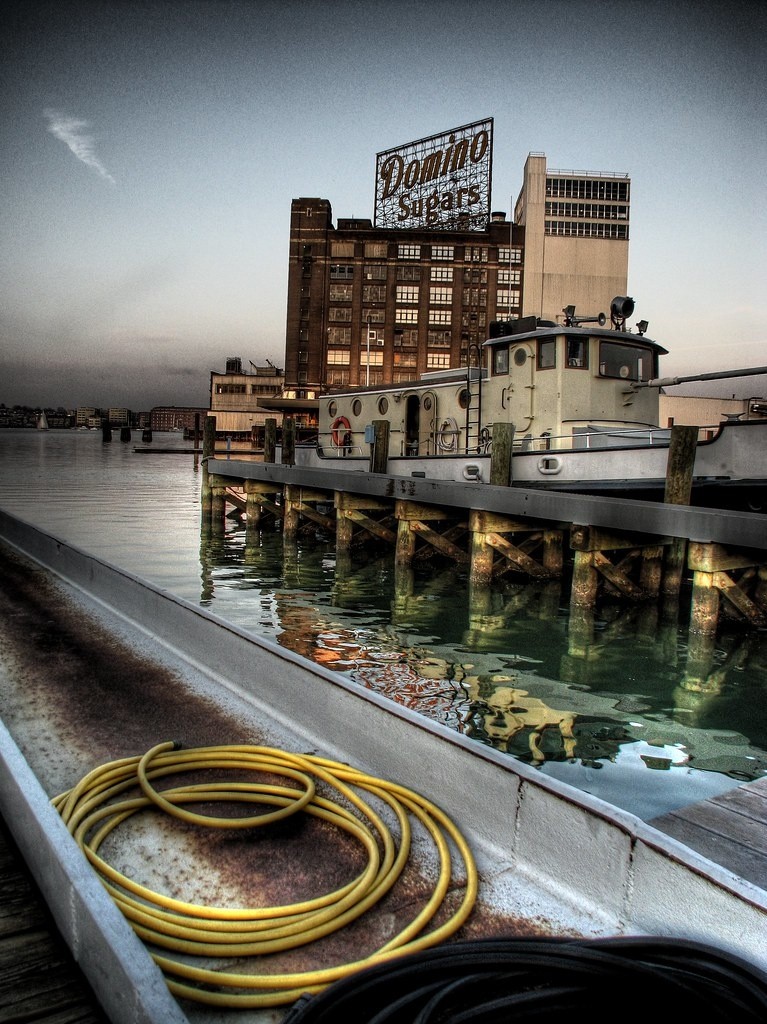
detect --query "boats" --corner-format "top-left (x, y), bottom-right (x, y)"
top-left (275, 297), bottom-right (767, 491)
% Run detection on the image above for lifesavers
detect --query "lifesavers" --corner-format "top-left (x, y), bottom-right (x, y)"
top-left (332, 415), bottom-right (351, 446)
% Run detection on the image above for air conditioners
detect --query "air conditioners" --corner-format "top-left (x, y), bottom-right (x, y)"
top-left (377, 339), bottom-right (385, 346)
top-left (445, 331), bottom-right (451, 336)
